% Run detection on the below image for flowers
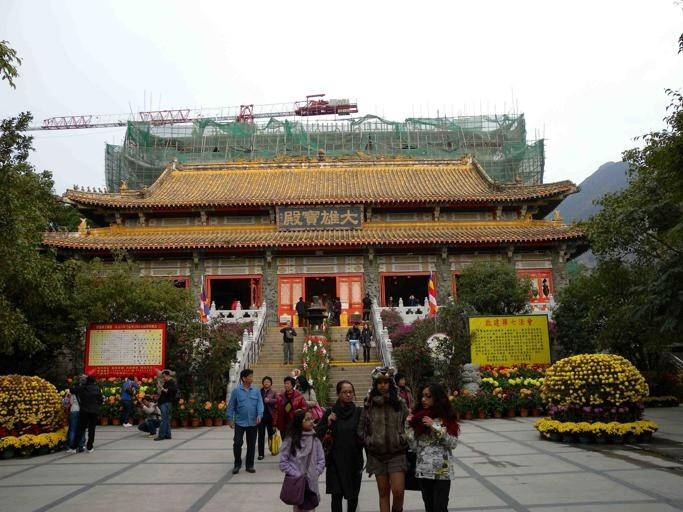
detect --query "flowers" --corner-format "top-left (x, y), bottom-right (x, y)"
top-left (449, 353), bottom-right (659, 437)
top-left (0, 374), bottom-right (226, 449)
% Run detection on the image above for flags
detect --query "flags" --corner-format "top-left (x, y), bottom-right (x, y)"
top-left (427, 272), bottom-right (438, 320)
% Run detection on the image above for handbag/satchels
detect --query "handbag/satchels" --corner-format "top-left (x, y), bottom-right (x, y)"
top-left (404, 438), bottom-right (422, 492)
top-left (280, 436), bottom-right (314, 506)
top-left (322, 405), bottom-right (332, 457)
top-left (271, 429), bottom-right (282, 457)
top-left (370, 336), bottom-right (376, 348)
top-left (126, 384), bottom-right (135, 396)
top-left (309, 388), bottom-right (325, 420)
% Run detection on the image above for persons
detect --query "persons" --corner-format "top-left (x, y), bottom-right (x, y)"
top-left (346, 322), bottom-right (373, 362)
top-left (363, 294), bottom-right (372, 322)
top-left (120, 375), bottom-right (141, 427)
top-left (136, 396), bottom-right (162, 438)
top-left (65, 393), bottom-right (81, 453)
top-left (69, 375), bottom-right (101, 454)
top-left (280, 320), bottom-right (297, 365)
top-left (226, 367), bottom-right (460, 511)
top-left (153, 370), bottom-right (176, 441)
top-left (296, 293), bottom-right (342, 325)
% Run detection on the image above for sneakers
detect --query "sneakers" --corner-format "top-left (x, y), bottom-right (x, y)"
top-left (123, 421), bottom-right (172, 441)
top-left (67, 446), bottom-right (94, 455)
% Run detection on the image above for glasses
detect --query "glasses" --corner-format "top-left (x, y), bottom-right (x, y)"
top-left (339, 391), bottom-right (354, 395)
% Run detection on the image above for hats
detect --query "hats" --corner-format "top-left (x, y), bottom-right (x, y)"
top-left (371, 365), bottom-right (398, 378)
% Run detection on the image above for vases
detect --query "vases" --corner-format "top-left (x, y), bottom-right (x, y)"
top-left (0, 447), bottom-right (48, 460)
top-left (541, 431), bottom-right (652, 445)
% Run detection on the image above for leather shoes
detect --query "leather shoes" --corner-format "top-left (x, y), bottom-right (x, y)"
top-left (258, 455), bottom-right (265, 460)
top-left (232, 461), bottom-right (242, 474)
top-left (246, 466), bottom-right (256, 473)
